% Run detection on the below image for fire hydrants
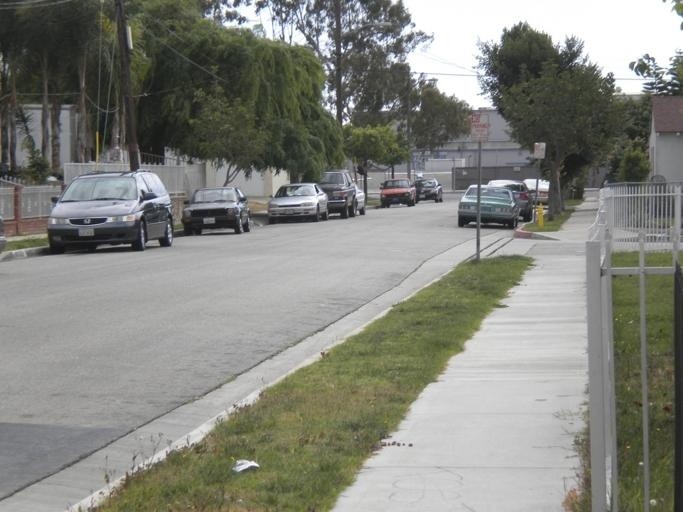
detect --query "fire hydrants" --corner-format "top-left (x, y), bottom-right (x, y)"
top-left (536, 202), bottom-right (544, 228)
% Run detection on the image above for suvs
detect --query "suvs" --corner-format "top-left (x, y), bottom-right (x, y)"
top-left (320, 169), bottom-right (358, 218)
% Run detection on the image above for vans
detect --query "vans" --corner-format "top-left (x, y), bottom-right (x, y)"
top-left (47, 169), bottom-right (173, 250)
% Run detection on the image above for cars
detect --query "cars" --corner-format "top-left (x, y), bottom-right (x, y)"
top-left (457, 184), bottom-right (519, 228)
top-left (181, 187), bottom-right (250, 233)
top-left (268, 181), bottom-right (329, 221)
top-left (415, 179), bottom-right (442, 202)
top-left (488, 179), bottom-right (536, 222)
top-left (354, 183), bottom-right (366, 215)
top-left (523, 179), bottom-right (550, 204)
top-left (379, 177), bottom-right (416, 206)
